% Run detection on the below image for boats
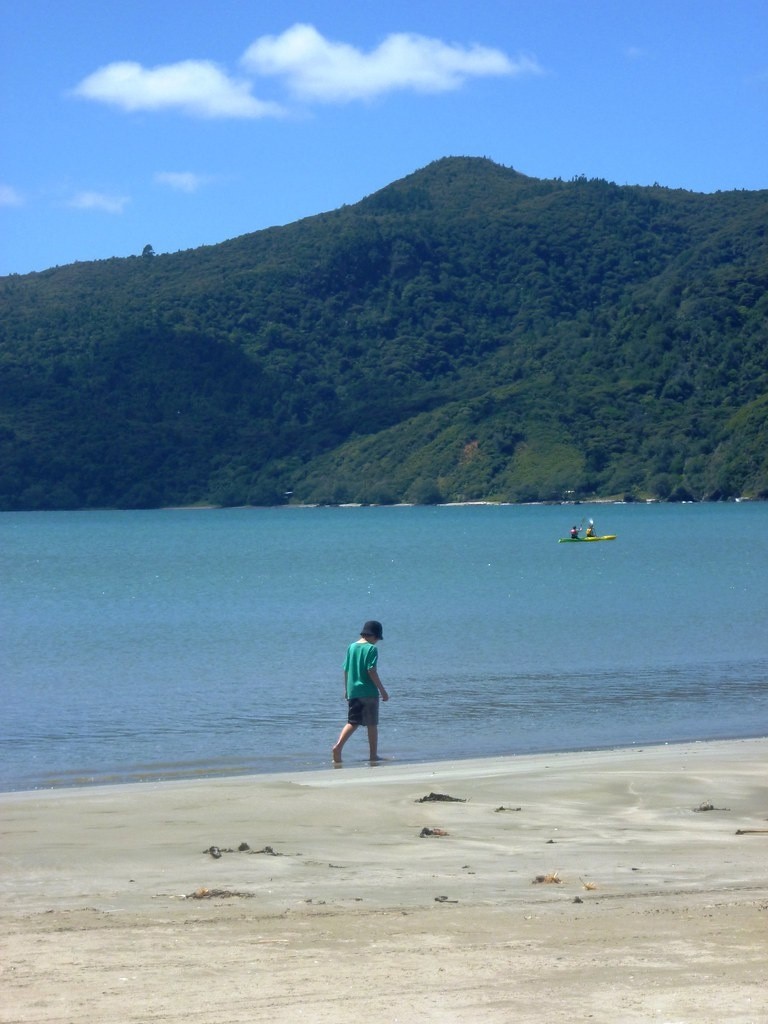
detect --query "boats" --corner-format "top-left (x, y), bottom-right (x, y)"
top-left (558, 533), bottom-right (618, 544)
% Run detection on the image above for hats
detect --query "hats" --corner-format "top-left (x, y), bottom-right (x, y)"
top-left (359, 620), bottom-right (384, 640)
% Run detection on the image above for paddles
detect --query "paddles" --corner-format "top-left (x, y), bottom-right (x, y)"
top-left (580, 516), bottom-right (585, 526)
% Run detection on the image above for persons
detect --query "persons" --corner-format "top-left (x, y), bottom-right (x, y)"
top-left (571, 526), bottom-right (579, 538)
top-left (333, 620), bottom-right (388, 761)
top-left (586, 527), bottom-right (594, 537)
